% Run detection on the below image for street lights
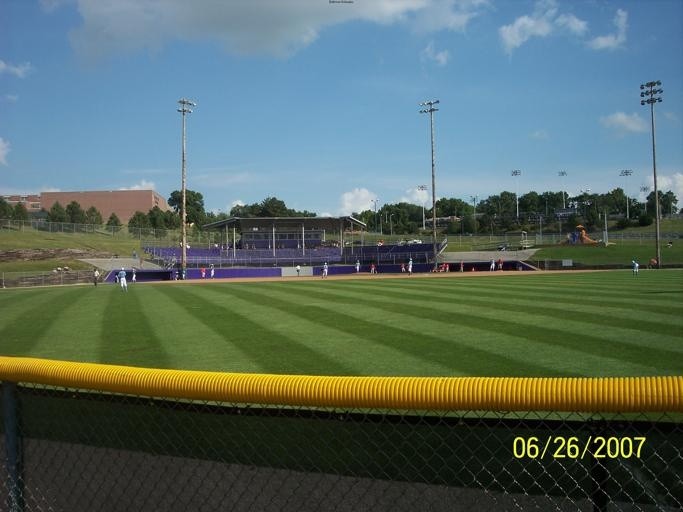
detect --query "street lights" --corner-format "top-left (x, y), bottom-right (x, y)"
top-left (175, 97), bottom-right (197, 279)
top-left (417, 99), bottom-right (440, 273)
top-left (640, 80), bottom-right (664, 268)
top-left (558, 167), bottom-right (567, 210)
top-left (469, 192), bottom-right (478, 235)
top-left (618, 169), bottom-right (635, 219)
top-left (510, 168), bottom-right (522, 217)
top-left (580, 185), bottom-right (590, 217)
top-left (370, 197), bottom-right (394, 236)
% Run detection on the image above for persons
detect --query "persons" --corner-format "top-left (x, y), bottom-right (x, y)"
top-left (200, 265), bottom-right (205, 278)
top-left (92, 268), bottom-right (99, 287)
top-left (647, 256), bottom-right (658, 269)
top-left (399, 262), bottom-right (405, 272)
top-left (630, 260), bottom-right (639, 278)
top-left (403, 238), bottom-right (410, 249)
top-left (459, 259), bottom-right (464, 273)
top-left (209, 263), bottom-right (214, 278)
top-left (117, 267), bottom-right (127, 293)
top-left (295, 263), bottom-right (300, 278)
top-left (354, 259), bottom-right (360, 273)
top-left (406, 258), bottom-right (412, 276)
top-left (173, 270), bottom-right (179, 282)
top-left (129, 268), bottom-right (136, 289)
top-left (488, 258), bottom-right (495, 271)
top-left (368, 262), bottom-right (376, 274)
top-left (320, 262), bottom-right (328, 279)
top-left (496, 258), bottom-right (503, 272)
top-left (666, 239), bottom-right (673, 248)
